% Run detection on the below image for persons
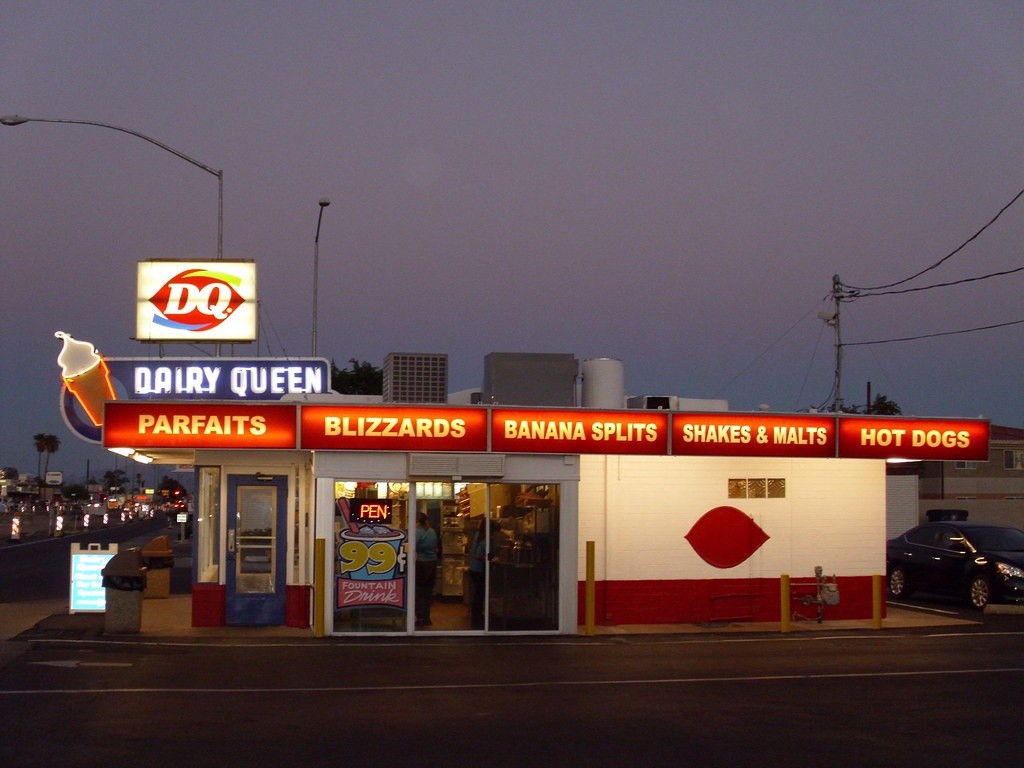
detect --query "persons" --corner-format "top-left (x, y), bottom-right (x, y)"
top-left (415, 511), bottom-right (437, 626)
top-left (470, 517), bottom-right (501, 621)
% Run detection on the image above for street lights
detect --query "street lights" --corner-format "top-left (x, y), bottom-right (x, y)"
top-left (0, 113), bottom-right (225, 359)
top-left (311, 196), bottom-right (331, 357)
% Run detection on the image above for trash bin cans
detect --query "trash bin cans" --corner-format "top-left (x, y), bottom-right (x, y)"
top-left (100, 547), bottom-right (148, 635)
top-left (142, 533), bottom-right (173, 600)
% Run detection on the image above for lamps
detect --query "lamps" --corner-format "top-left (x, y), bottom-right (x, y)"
top-left (108, 447), bottom-right (135, 457)
top-left (130, 452), bottom-right (153, 464)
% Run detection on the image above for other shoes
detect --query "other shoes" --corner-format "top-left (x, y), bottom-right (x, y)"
top-left (416, 617), bottom-right (432, 626)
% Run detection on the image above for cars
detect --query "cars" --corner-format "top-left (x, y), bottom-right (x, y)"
top-left (886, 520), bottom-right (1024, 612)
